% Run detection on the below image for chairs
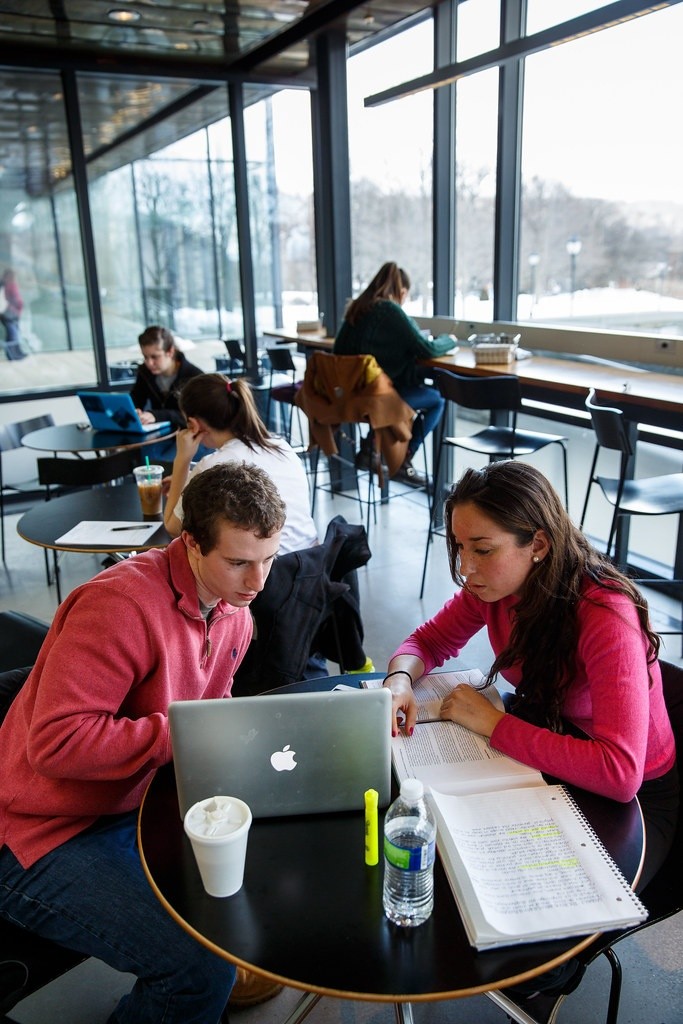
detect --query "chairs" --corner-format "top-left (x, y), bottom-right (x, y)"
top-left (0, 339), bottom-right (683, 1024)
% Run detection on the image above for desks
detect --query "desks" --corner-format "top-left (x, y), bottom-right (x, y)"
top-left (263, 329), bottom-right (683, 578)
top-left (16, 486), bottom-right (174, 606)
top-left (22, 421), bottom-right (178, 486)
top-left (138, 672), bottom-right (647, 1024)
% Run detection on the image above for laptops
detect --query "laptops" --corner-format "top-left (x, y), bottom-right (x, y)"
top-left (77, 392), bottom-right (170, 433)
top-left (168, 687), bottom-right (392, 820)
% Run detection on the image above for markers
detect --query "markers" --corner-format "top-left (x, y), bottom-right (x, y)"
top-left (363, 789), bottom-right (379, 866)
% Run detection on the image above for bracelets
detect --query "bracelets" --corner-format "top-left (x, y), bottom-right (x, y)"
top-left (382, 670), bottom-right (413, 685)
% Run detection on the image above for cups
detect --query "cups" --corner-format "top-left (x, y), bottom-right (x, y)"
top-left (133, 465), bottom-right (164, 515)
top-left (183, 796), bottom-right (253, 899)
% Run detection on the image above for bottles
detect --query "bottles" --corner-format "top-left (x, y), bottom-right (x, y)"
top-left (382, 778), bottom-right (437, 927)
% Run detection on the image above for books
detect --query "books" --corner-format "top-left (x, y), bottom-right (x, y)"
top-left (359, 668), bottom-right (547, 796)
top-left (425, 785), bottom-right (649, 950)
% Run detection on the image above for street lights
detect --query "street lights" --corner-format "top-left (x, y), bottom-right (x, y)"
top-left (527, 249), bottom-right (542, 294)
top-left (565, 237), bottom-right (581, 293)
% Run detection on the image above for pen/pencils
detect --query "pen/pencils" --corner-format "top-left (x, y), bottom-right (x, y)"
top-left (449, 322), bottom-right (459, 334)
top-left (109, 524), bottom-right (152, 531)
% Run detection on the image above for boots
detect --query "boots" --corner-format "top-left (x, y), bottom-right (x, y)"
top-left (392, 455), bottom-right (427, 486)
top-left (354, 437), bottom-right (389, 475)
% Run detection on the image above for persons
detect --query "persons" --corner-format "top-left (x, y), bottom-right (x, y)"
top-left (0, 268), bottom-right (27, 360)
top-left (332, 262), bottom-right (458, 486)
top-left (0, 462), bottom-right (287, 1024)
top-left (128, 326), bottom-right (215, 463)
top-left (383, 460), bottom-right (676, 1024)
top-left (164, 373), bottom-right (318, 561)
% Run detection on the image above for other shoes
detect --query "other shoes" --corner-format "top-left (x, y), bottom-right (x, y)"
top-left (228, 964), bottom-right (283, 1005)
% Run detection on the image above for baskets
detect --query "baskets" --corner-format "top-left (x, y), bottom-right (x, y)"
top-left (475, 343), bottom-right (517, 365)
